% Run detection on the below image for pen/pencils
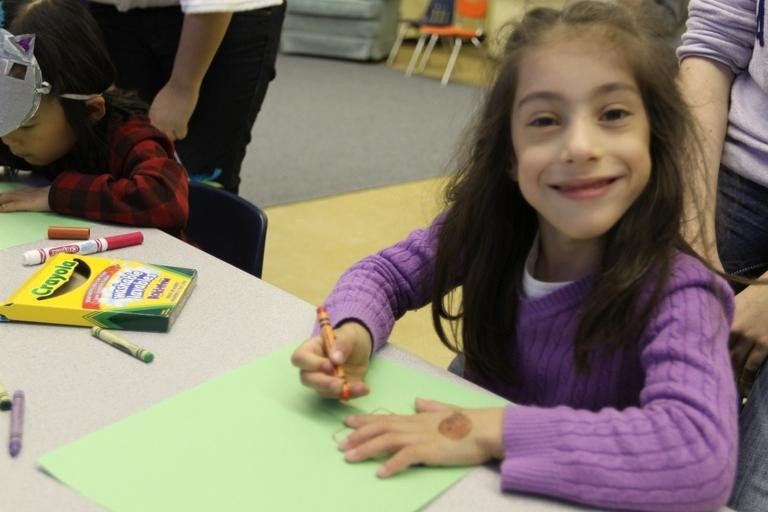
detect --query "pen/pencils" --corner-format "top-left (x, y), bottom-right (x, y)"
top-left (316, 305), bottom-right (352, 401)
top-left (22, 231), bottom-right (144, 267)
top-left (91, 325), bottom-right (154, 362)
top-left (0, 382), bottom-right (22, 456)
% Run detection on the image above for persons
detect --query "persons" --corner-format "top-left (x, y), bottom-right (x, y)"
top-left (666, 1), bottom-right (768, 511)
top-left (285, 2), bottom-right (742, 509)
top-left (89, 1), bottom-right (288, 200)
top-left (0, 0), bottom-right (195, 248)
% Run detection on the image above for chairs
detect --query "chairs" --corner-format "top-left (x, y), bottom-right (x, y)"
top-left (182, 180), bottom-right (267, 280)
top-left (387, 0), bottom-right (492, 86)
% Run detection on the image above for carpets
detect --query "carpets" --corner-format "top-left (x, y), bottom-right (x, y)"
top-left (245, 172), bottom-right (473, 376)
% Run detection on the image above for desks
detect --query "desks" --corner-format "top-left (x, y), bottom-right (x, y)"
top-left (1, 211), bottom-right (573, 512)
